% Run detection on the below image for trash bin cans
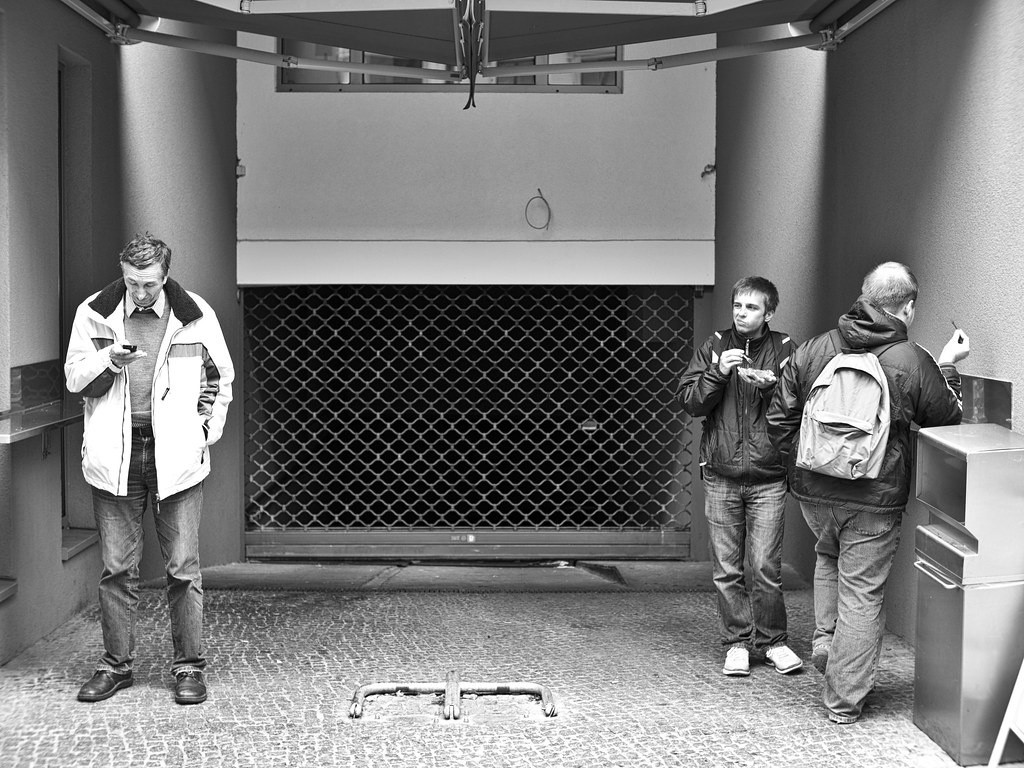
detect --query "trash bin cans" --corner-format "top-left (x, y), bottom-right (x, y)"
top-left (911, 423), bottom-right (1023, 767)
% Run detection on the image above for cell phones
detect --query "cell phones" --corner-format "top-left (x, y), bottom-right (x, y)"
top-left (122, 345), bottom-right (137, 352)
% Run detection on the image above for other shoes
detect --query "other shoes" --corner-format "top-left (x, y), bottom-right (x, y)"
top-left (811, 646), bottom-right (832, 674)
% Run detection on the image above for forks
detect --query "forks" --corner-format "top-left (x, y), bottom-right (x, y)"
top-left (743, 355), bottom-right (753, 364)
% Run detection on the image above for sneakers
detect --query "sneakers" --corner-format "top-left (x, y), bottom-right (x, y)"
top-left (723, 646), bottom-right (750, 676)
top-left (765, 643), bottom-right (803, 675)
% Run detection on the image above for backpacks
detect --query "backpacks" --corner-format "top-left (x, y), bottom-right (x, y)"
top-left (795, 329), bottom-right (907, 479)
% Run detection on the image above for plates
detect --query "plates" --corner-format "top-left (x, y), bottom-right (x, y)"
top-left (737, 367), bottom-right (774, 378)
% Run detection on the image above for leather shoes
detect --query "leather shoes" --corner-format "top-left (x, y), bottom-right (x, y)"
top-left (176, 671), bottom-right (206, 703)
top-left (78, 670), bottom-right (133, 701)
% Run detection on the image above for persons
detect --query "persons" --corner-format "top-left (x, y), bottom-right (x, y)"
top-left (766, 261), bottom-right (971, 724)
top-left (675, 277), bottom-right (803, 675)
top-left (64, 233), bottom-right (234, 704)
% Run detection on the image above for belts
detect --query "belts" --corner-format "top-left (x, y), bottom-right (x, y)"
top-left (132, 426), bottom-right (153, 437)
